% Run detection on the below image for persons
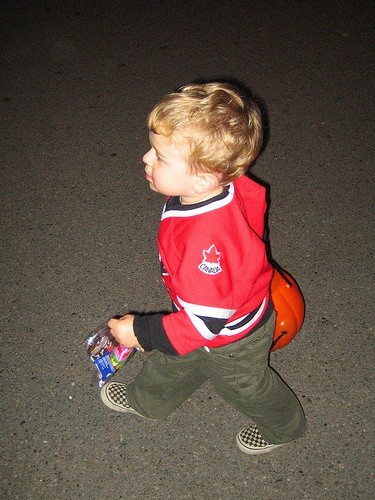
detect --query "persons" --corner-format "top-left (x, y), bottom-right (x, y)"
top-left (100, 83), bottom-right (308, 455)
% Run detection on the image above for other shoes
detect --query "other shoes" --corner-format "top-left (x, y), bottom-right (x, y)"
top-left (100, 381), bottom-right (159, 421)
top-left (236, 424), bottom-right (302, 455)
